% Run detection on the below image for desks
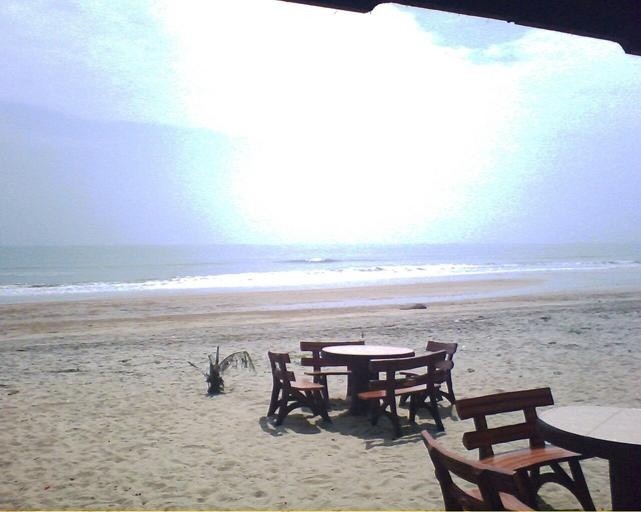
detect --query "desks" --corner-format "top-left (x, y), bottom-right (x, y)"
top-left (536, 405), bottom-right (640, 512)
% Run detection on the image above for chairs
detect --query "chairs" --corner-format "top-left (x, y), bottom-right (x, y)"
top-left (452, 387), bottom-right (598, 510)
top-left (267, 340), bottom-right (458, 437)
top-left (420, 427), bottom-right (555, 510)
